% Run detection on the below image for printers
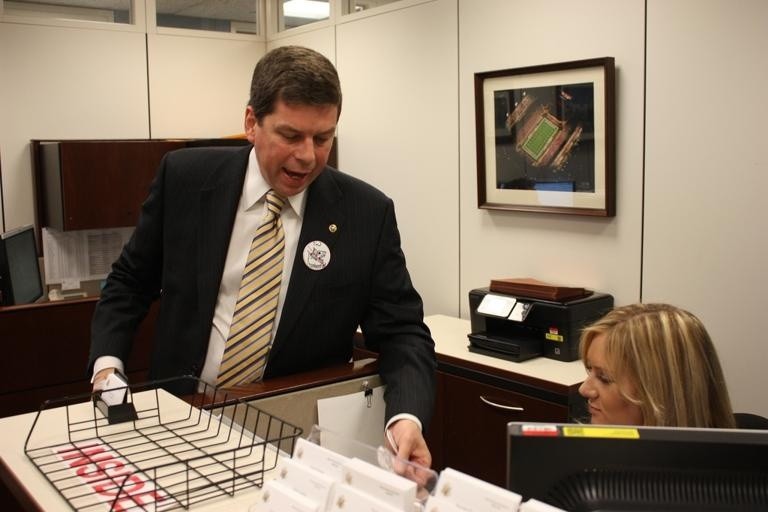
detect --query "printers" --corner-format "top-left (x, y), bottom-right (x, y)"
top-left (467, 286), bottom-right (615, 363)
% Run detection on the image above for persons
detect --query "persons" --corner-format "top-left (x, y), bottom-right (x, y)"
top-left (88, 45), bottom-right (435, 490)
top-left (578, 303), bottom-right (738, 429)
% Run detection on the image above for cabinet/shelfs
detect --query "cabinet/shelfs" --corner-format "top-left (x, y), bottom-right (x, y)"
top-left (29, 135), bottom-right (339, 235)
top-left (352, 313), bottom-right (592, 492)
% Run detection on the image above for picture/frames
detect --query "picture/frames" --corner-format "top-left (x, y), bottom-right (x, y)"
top-left (474, 56), bottom-right (616, 219)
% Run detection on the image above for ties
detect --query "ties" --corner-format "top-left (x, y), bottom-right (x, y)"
top-left (215, 190), bottom-right (287, 387)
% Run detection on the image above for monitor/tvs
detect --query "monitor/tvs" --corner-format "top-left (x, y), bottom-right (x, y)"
top-left (503, 420), bottom-right (766, 509)
top-left (0, 224), bottom-right (46, 305)
top-left (529, 183), bottom-right (578, 192)
